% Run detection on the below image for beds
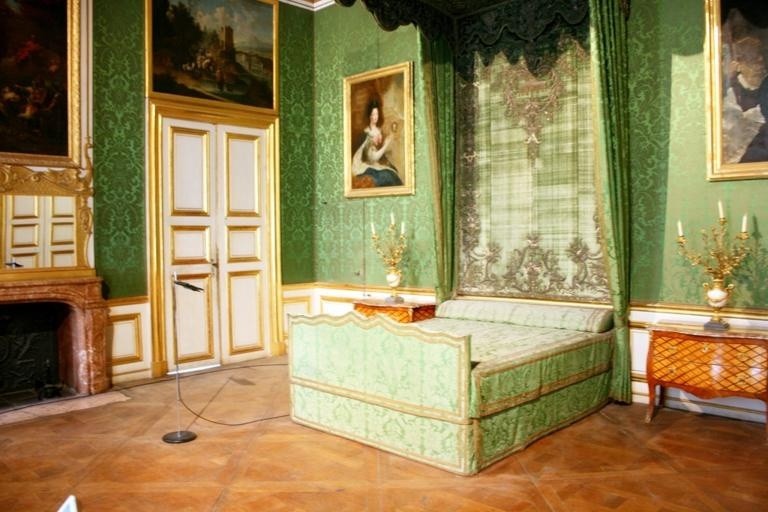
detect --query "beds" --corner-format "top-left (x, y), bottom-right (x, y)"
top-left (286, 290), bottom-right (618, 475)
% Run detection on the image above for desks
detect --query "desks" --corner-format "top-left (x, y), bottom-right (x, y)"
top-left (643, 324), bottom-right (767, 426)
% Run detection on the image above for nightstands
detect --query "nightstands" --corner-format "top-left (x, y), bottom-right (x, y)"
top-left (353, 302), bottom-right (435, 324)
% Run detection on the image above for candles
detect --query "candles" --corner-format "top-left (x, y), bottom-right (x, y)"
top-left (740, 212), bottom-right (748, 234)
top-left (676, 218), bottom-right (683, 237)
top-left (400, 222), bottom-right (404, 233)
top-left (389, 212), bottom-right (395, 225)
top-left (371, 222), bottom-right (375, 234)
top-left (717, 200), bottom-right (725, 219)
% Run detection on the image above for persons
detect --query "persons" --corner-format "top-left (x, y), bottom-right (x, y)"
top-left (352, 101), bottom-right (403, 186)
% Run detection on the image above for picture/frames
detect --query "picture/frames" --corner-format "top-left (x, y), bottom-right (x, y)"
top-left (142, 0), bottom-right (279, 116)
top-left (1, 0), bottom-right (81, 170)
top-left (703, 0), bottom-right (767, 183)
top-left (342, 60), bottom-right (414, 198)
